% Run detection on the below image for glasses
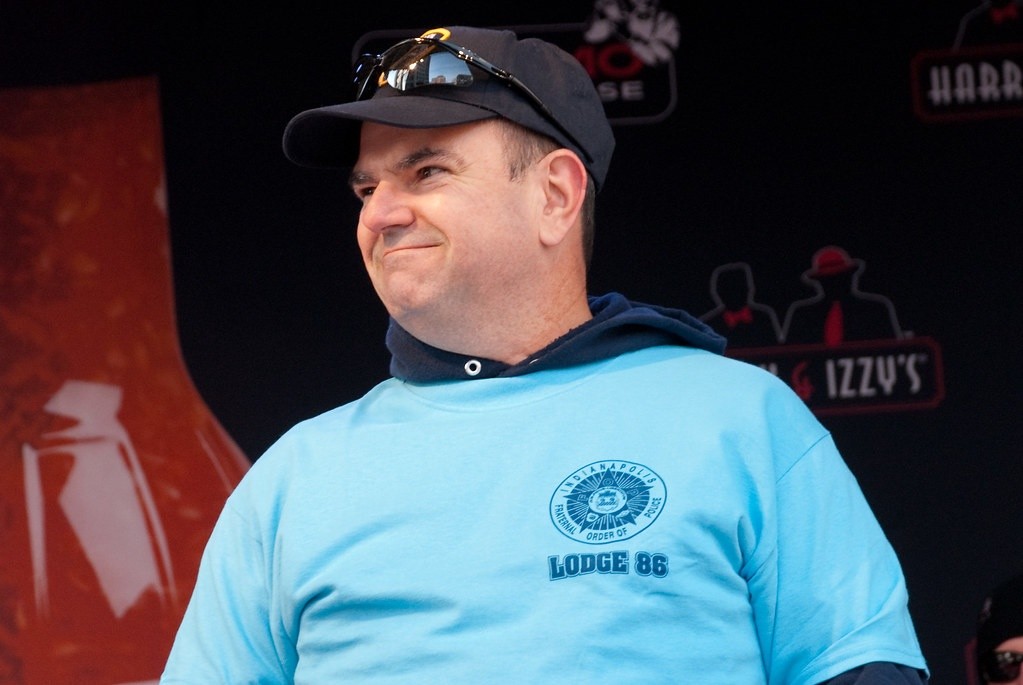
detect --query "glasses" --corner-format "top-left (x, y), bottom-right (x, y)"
top-left (346, 36), bottom-right (595, 162)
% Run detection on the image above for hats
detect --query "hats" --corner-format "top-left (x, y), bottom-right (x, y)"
top-left (282, 27), bottom-right (617, 196)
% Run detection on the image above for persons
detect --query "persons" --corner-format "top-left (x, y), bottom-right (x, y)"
top-left (149, 24), bottom-right (930, 683)
